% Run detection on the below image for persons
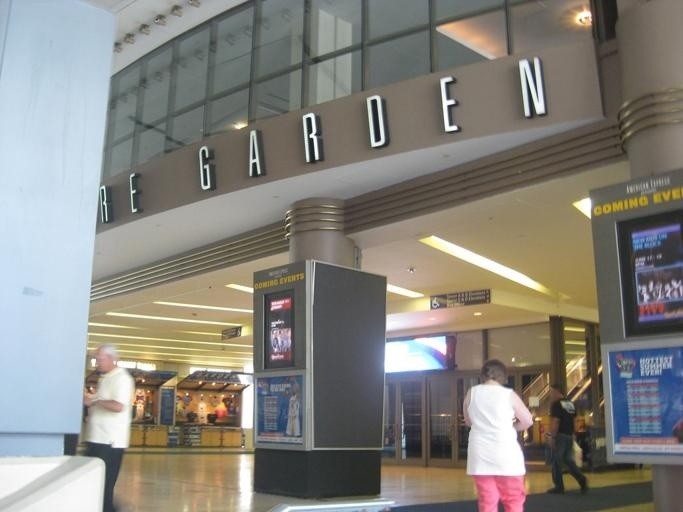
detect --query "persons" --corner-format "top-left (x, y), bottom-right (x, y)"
top-left (545, 384), bottom-right (591, 494)
top-left (270, 327), bottom-right (290, 354)
top-left (282, 375), bottom-right (302, 438)
top-left (82, 343), bottom-right (135, 512)
top-left (461, 358), bottom-right (535, 511)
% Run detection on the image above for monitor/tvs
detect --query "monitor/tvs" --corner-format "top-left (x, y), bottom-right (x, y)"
top-left (207, 414), bottom-right (217, 423)
top-left (385, 335), bottom-right (457, 375)
top-left (616, 209), bottom-right (683, 338)
top-left (264, 289), bottom-right (294, 369)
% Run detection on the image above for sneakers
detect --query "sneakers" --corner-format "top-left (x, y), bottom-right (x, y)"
top-left (580, 480), bottom-right (590, 495)
top-left (548, 487), bottom-right (565, 494)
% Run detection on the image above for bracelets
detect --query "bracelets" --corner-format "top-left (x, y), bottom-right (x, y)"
top-left (90, 396), bottom-right (98, 406)
top-left (545, 432), bottom-right (554, 437)
